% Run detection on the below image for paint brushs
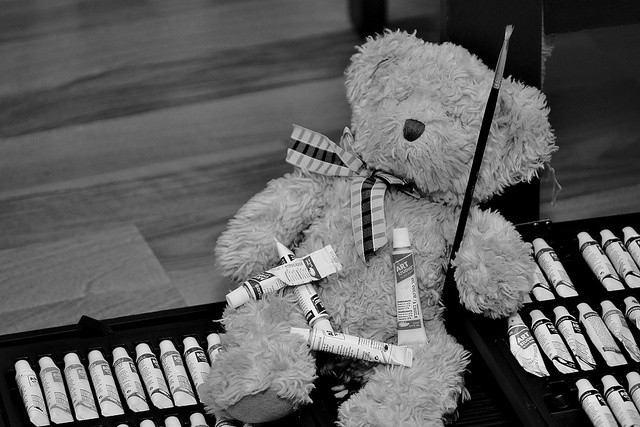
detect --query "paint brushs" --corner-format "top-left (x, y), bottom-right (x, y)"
top-left (443, 25), bottom-right (514, 298)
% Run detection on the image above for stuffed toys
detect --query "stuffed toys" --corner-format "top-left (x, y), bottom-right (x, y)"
top-left (205, 25), bottom-right (560, 427)
